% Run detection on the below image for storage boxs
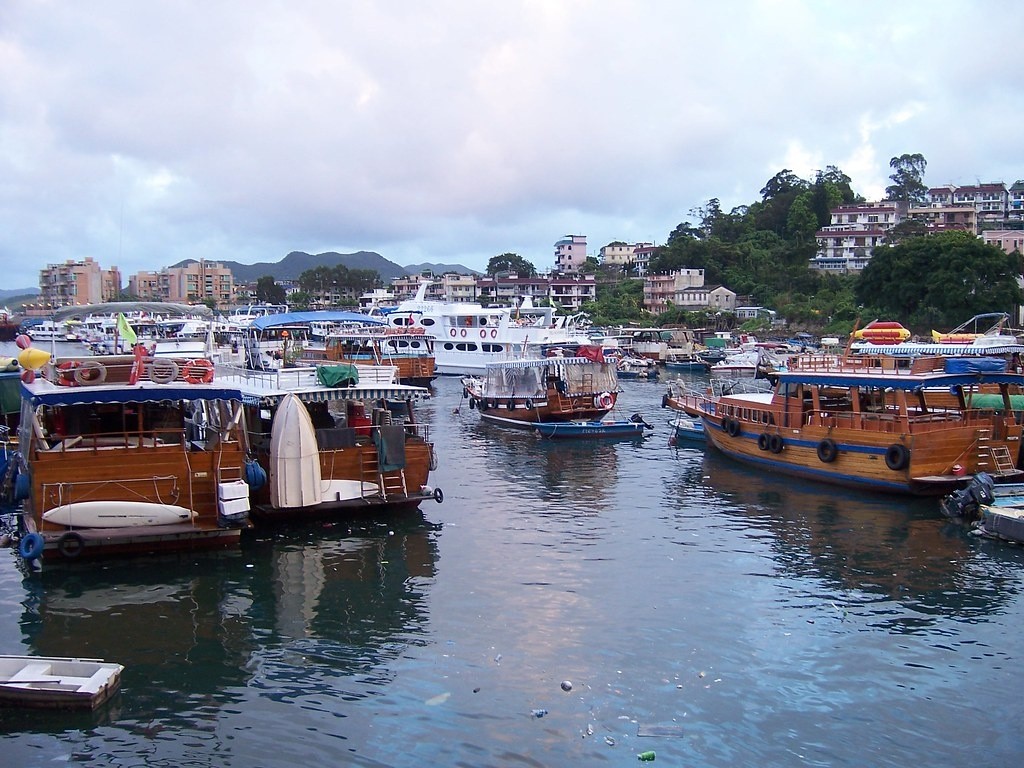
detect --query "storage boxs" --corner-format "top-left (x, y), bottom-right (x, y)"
top-left (219, 482), bottom-right (251, 514)
top-left (350, 417), bottom-right (371, 436)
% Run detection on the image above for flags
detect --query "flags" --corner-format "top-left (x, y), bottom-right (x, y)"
top-left (118, 314), bottom-right (138, 344)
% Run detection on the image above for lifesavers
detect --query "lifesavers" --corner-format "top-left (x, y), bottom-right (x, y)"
top-left (461, 329), bottom-right (466, 337)
top-left (491, 330), bottom-right (498, 338)
top-left (450, 329), bottom-right (456, 336)
top-left (479, 329), bottom-right (486, 338)
top-left (950, 383), bottom-right (960, 393)
top-left (60, 534), bottom-right (85, 556)
top-left (147, 358), bottom-right (178, 381)
top-left (75, 360), bottom-right (107, 385)
top-left (20, 534), bottom-right (46, 558)
top-left (720, 415), bottom-right (908, 471)
top-left (181, 359), bottom-right (213, 383)
top-left (55, 362), bottom-right (87, 385)
top-left (385, 326), bottom-right (423, 335)
top-left (600, 391), bottom-right (615, 409)
top-left (435, 488), bottom-right (443, 502)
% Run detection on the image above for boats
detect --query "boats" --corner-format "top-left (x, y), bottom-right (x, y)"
top-left (184, 310), bottom-right (442, 516)
top-left (851, 322), bottom-right (911, 345)
top-left (14, 301), bottom-right (252, 562)
top-left (530, 421), bottom-right (647, 439)
top-left (663, 372), bottom-right (1024, 498)
top-left (19, 280), bottom-right (818, 414)
top-left (759, 340), bottom-right (1024, 407)
top-left (669, 418), bottom-right (706, 441)
top-left (458, 354), bottom-right (619, 428)
top-left (931, 328), bottom-right (984, 345)
